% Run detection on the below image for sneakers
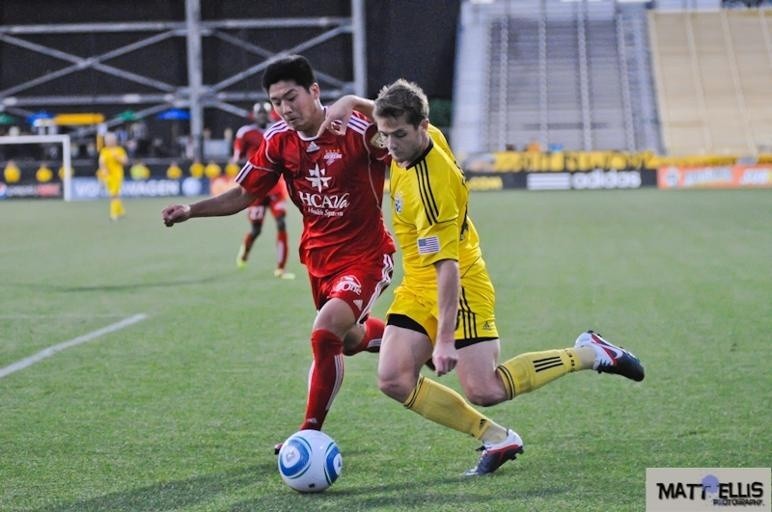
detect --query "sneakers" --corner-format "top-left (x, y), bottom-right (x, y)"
top-left (272, 268), bottom-right (297, 281)
top-left (455, 425), bottom-right (526, 478)
top-left (235, 258), bottom-right (253, 271)
top-left (272, 442), bottom-right (282, 456)
top-left (424, 355), bottom-right (438, 373)
top-left (573, 327), bottom-right (646, 385)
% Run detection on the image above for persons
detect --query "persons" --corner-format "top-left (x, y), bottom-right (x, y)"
top-left (233, 100), bottom-right (295, 280)
top-left (161, 55), bottom-right (435, 455)
top-left (316, 78), bottom-right (646, 478)
top-left (0, 121), bottom-right (235, 159)
top-left (97, 130), bottom-right (128, 220)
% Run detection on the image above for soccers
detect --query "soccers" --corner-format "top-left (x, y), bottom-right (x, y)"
top-left (278, 430), bottom-right (342, 493)
